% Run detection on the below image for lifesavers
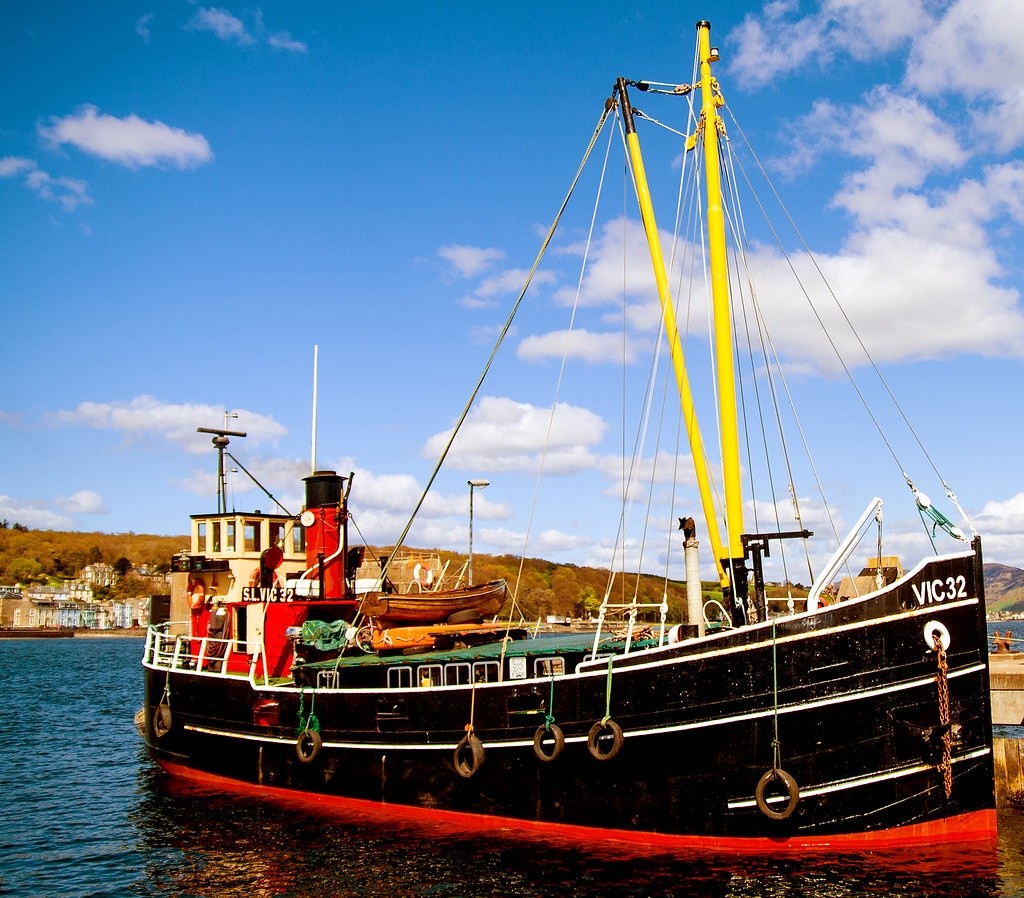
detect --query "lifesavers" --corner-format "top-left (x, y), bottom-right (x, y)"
top-left (755, 768), bottom-right (799, 820)
top-left (248, 567), bottom-right (260, 587)
top-left (153, 704), bottom-right (172, 737)
top-left (454, 734), bottom-right (484, 777)
top-left (296, 730), bottom-right (321, 762)
top-left (588, 721), bottom-right (624, 760)
top-left (447, 608), bottom-right (481, 625)
top-left (413, 562), bottom-right (433, 587)
top-left (187, 578), bottom-right (205, 611)
top-left (533, 723), bottom-right (564, 762)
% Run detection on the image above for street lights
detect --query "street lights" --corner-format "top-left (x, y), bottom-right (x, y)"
top-left (466, 480), bottom-right (490, 586)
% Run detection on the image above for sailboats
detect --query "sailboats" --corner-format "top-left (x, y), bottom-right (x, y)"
top-left (134, 18), bottom-right (999, 857)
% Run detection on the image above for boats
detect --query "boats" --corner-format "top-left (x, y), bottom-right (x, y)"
top-left (357, 577), bottom-right (511, 623)
